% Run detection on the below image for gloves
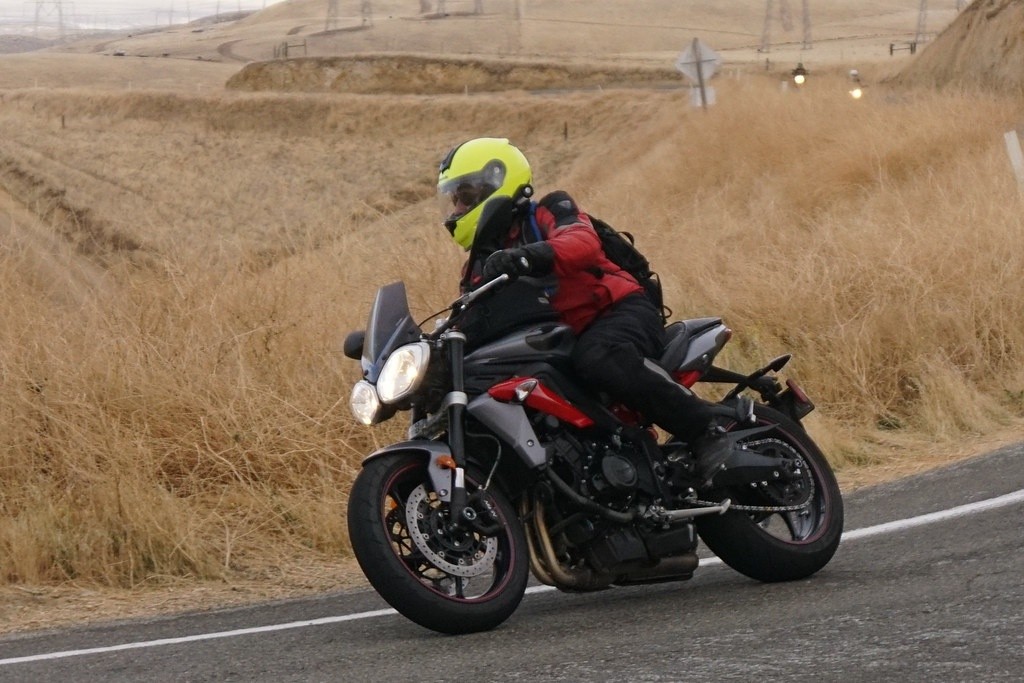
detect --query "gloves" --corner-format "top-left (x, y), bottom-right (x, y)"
top-left (479, 239), bottom-right (554, 282)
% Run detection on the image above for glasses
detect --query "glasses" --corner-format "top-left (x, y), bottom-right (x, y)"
top-left (449, 187), bottom-right (485, 205)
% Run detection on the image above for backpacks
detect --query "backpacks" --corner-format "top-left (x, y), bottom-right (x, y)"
top-left (584, 212), bottom-right (668, 322)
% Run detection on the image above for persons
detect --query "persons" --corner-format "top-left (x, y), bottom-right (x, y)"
top-left (792, 63), bottom-right (809, 76)
top-left (434, 137), bottom-right (734, 483)
top-left (847, 70), bottom-right (861, 83)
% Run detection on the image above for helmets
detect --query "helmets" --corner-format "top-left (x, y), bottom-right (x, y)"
top-left (440, 136), bottom-right (533, 252)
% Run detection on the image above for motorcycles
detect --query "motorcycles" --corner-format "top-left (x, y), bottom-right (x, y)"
top-left (344, 269), bottom-right (845, 634)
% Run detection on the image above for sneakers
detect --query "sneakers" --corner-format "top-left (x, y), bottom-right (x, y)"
top-left (689, 418), bottom-right (734, 488)
